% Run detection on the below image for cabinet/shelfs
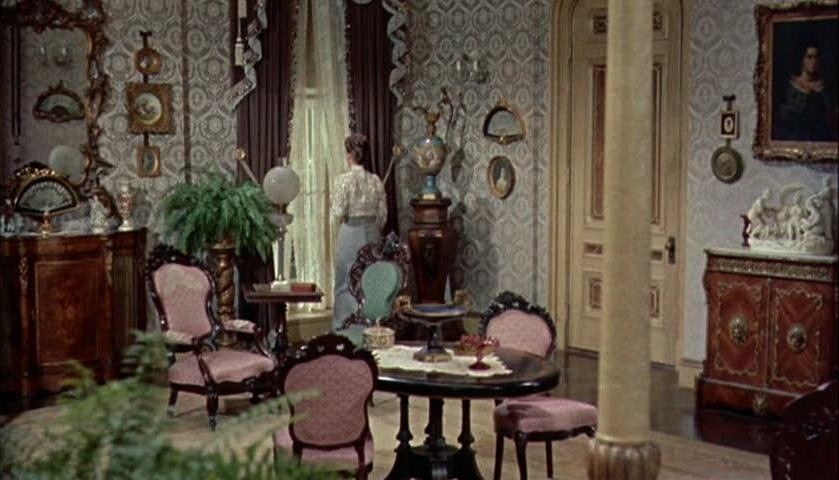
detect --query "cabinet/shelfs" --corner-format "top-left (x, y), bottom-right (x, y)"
top-left (0, 223), bottom-right (151, 418)
top-left (692, 243), bottom-right (839, 419)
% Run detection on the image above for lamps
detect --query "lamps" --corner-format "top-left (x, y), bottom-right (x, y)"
top-left (260, 163), bottom-right (302, 280)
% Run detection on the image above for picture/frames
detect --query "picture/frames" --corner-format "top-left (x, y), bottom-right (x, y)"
top-left (752, 2), bottom-right (839, 163)
top-left (121, 45), bottom-right (176, 179)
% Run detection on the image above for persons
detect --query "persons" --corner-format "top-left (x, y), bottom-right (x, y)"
top-left (333, 132), bottom-right (390, 320)
top-left (496, 164), bottom-right (508, 193)
top-left (778, 35), bottom-right (834, 141)
